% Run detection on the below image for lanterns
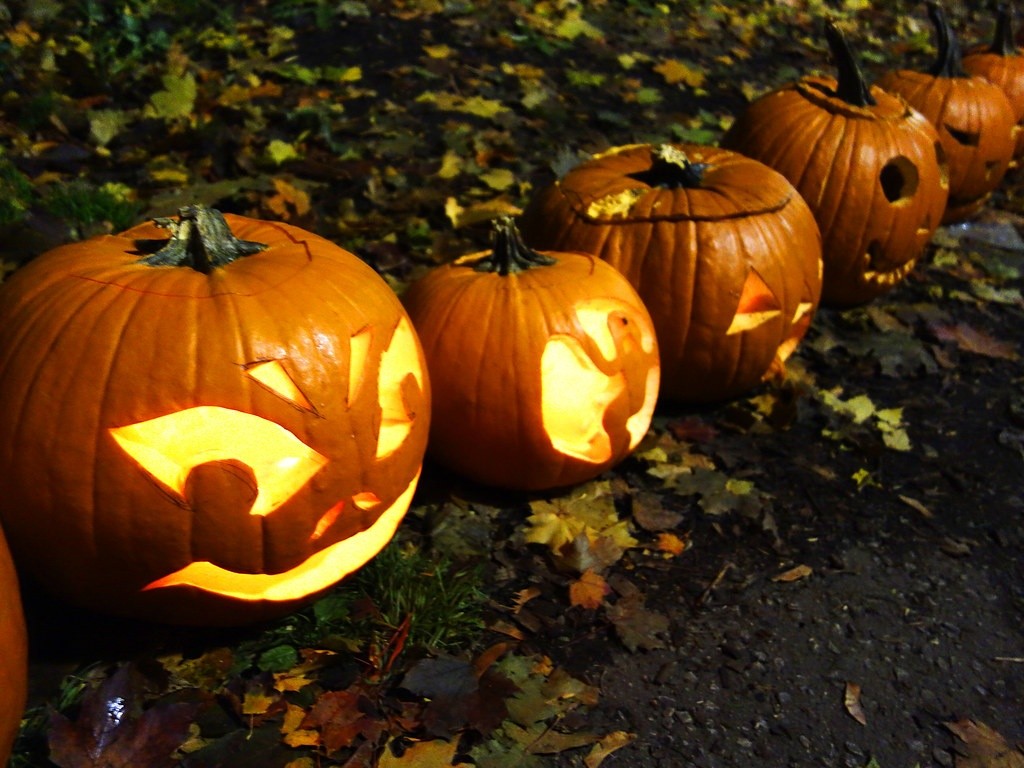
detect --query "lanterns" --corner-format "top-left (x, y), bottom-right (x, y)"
top-left (0, 2), bottom-right (1024, 768)
